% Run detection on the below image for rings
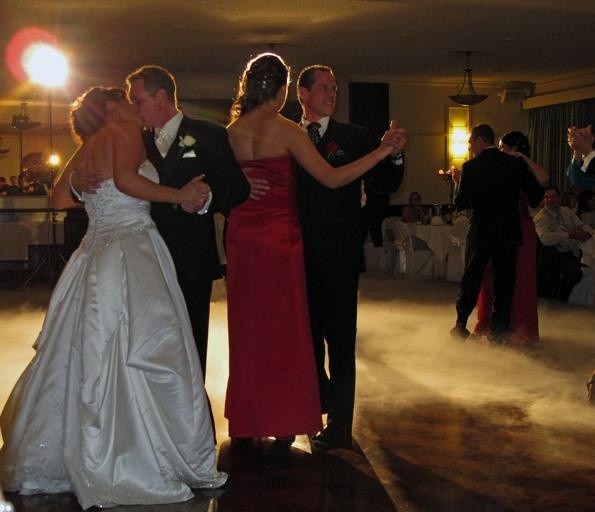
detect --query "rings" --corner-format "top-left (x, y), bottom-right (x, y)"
top-left (200, 194), bottom-right (205, 203)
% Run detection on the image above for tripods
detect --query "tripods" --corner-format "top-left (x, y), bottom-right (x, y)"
top-left (22, 90), bottom-right (67, 294)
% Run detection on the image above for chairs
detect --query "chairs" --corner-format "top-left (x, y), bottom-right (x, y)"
top-left (364, 216), bottom-right (471, 286)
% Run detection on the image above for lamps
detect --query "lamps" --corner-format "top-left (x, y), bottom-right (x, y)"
top-left (446, 50), bottom-right (488, 106)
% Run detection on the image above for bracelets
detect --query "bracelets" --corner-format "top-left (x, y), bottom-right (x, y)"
top-left (175, 189), bottom-right (179, 208)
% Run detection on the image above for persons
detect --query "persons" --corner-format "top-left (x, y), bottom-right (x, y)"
top-left (222, 52), bottom-right (403, 466)
top-left (68, 64), bottom-right (250, 446)
top-left (530, 184), bottom-right (592, 304)
top-left (0, 172), bottom-right (48, 195)
top-left (566, 123), bottom-right (595, 404)
top-left (0, 87), bottom-right (230, 510)
top-left (241, 63), bottom-right (408, 444)
top-left (401, 191), bottom-right (431, 226)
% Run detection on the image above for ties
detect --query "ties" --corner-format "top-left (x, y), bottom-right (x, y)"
top-left (307, 122), bottom-right (322, 146)
top-left (156, 129), bottom-right (168, 159)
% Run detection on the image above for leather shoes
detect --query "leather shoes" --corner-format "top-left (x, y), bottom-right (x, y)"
top-left (312, 426), bottom-right (352, 444)
top-left (450, 327), bottom-right (469, 340)
